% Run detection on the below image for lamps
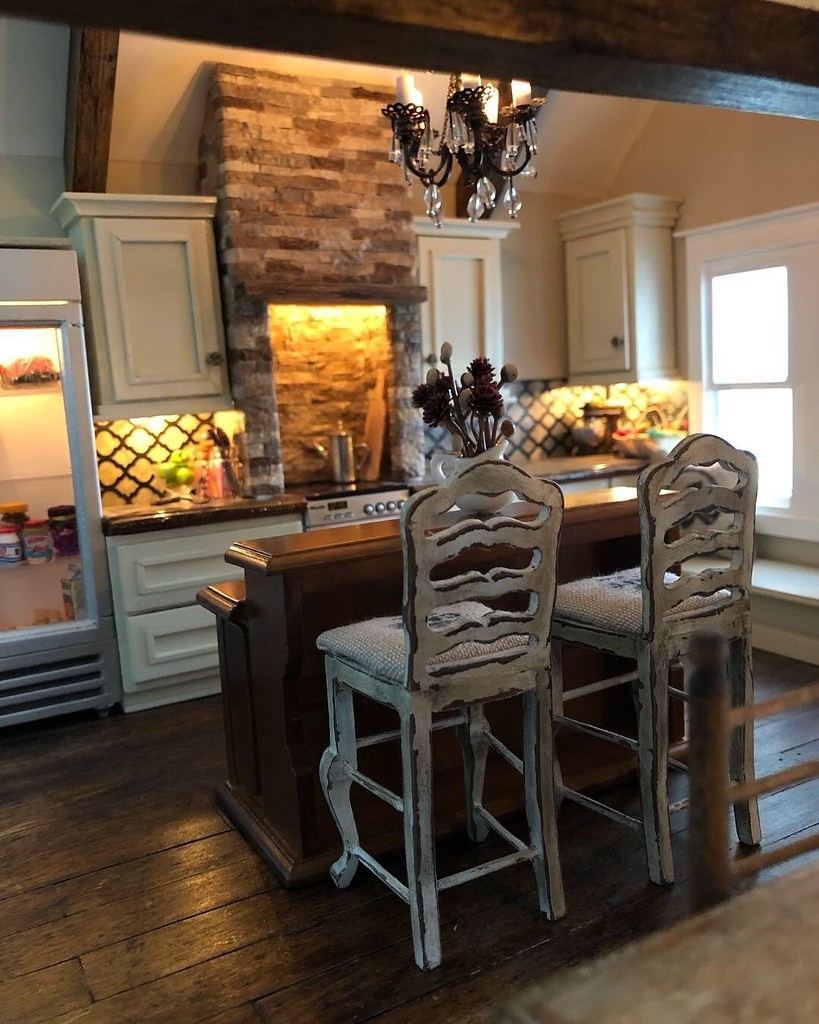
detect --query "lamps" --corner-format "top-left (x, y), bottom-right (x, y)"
top-left (381, 73), bottom-right (549, 229)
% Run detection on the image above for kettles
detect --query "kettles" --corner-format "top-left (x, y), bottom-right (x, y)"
top-left (312, 421), bottom-right (370, 484)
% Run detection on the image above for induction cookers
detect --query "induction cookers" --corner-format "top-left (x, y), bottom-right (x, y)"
top-left (283, 479), bottom-right (409, 526)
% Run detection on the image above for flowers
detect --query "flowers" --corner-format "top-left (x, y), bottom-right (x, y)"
top-left (411, 341), bottom-right (519, 455)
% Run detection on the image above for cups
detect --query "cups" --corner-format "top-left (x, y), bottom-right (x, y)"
top-left (212, 447), bottom-right (241, 499)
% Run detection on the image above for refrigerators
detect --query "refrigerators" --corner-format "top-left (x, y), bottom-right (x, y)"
top-left (0, 247), bottom-right (124, 734)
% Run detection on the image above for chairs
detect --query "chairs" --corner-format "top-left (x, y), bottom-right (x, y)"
top-left (551, 434), bottom-right (759, 888)
top-left (319, 459), bottom-right (565, 971)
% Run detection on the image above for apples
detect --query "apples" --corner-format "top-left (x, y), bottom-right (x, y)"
top-left (156, 450), bottom-right (195, 488)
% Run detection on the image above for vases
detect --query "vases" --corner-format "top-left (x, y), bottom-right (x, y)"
top-left (430, 440), bottom-right (516, 513)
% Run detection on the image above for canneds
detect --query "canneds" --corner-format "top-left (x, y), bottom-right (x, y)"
top-left (0, 502), bottom-right (77, 566)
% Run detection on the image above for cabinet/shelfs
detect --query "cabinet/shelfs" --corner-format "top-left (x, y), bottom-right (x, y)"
top-left (103, 494), bottom-right (309, 715)
top-left (410, 212), bottom-right (521, 388)
top-left (48, 191), bottom-right (233, 418)
top-left (553, 192), bottom-right (686, 384)
